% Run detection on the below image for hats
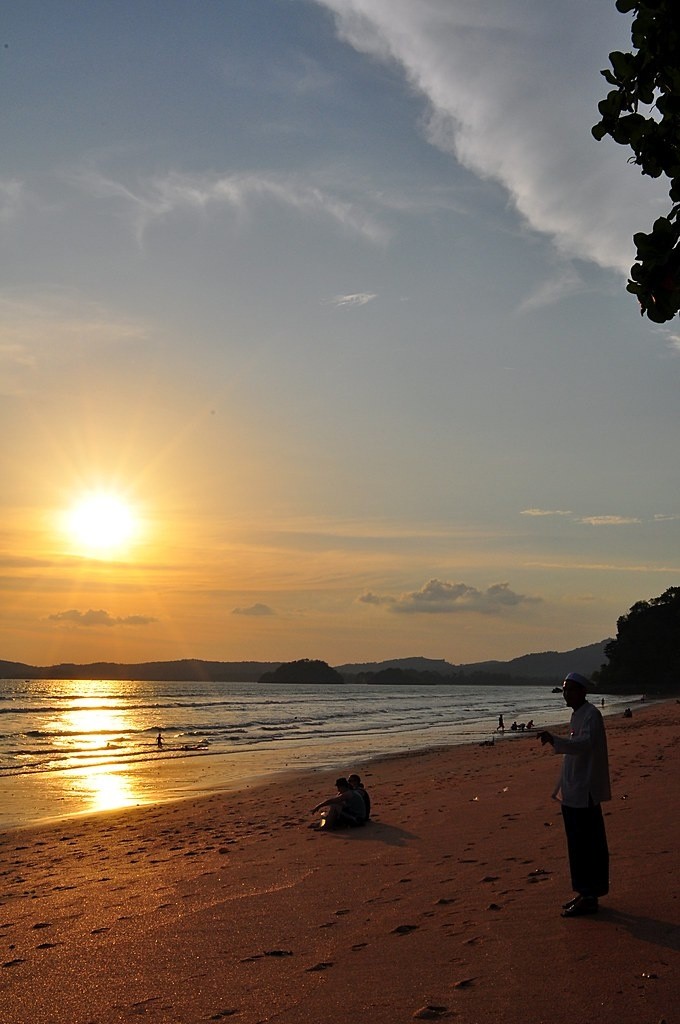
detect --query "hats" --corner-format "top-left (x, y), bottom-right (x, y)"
top-left (564, 672), bottom-right (591, 690)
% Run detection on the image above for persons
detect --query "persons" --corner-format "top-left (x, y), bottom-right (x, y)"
top-left (155, 733), bottom-right (200, 753)
top-left (600, 686), bottom-right (680, 719)
top-left (308, 773), bottom-right (371, 832)
top-left (533, 672), bottom-right (612, 918)
top-left (497, 712), bottom-right (535, 733)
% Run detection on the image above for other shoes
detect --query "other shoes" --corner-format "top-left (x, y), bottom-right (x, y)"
top-left (560, 893), bottom-right (584, 909)
top-left (314, 826), bottom-right (326, 831)
top-left (560, 899), bottom-right (599, 917)
